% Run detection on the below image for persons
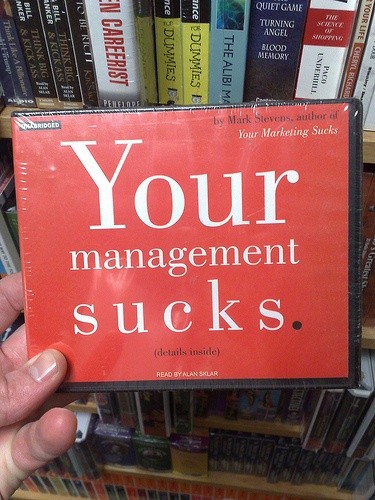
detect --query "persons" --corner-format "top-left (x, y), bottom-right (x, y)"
top-left (0, 269), bottom-right (78, 500)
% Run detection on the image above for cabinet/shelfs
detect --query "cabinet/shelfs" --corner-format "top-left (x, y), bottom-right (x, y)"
top-left (0, 105), bottom-right (375, 500)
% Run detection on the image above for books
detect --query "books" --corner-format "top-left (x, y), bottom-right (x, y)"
top-left (0, 0), bottom-right (375, 500)
